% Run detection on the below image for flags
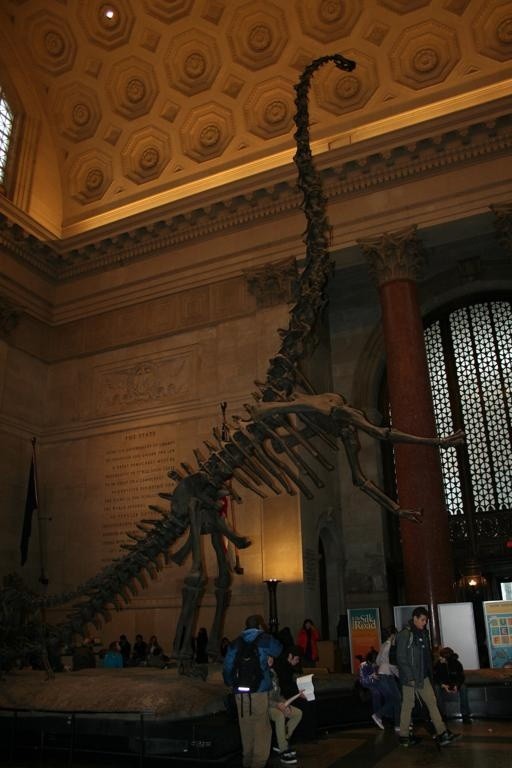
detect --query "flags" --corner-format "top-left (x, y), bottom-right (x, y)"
top-left (17, 454), bottom-right (40, 566)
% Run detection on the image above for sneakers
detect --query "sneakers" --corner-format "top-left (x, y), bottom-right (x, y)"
top-left (371, 713), bottom-right (385, 729)
top-left (273, 744), bottom-right (298, 764)
top-left (463, 715), bottom-right (472, 723)
top-left (399, 737), bottom-right (425, 747)
top-left (438, 730), bottom-right (463, 746)
top-left (395, 726), bottom-right (413, 731)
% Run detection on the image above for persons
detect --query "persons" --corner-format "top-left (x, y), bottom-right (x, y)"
top-left (57, 633), bottom-right (165, 670)
top-left (221, 615), bottom-right (322, 768)
top-left (358, 607), bottom-right (466, 745)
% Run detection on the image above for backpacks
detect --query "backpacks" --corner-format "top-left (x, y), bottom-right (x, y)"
top-left (233, 632), bottom-right (269, 693)
top-left (389, 626), bottom-right (413, 664)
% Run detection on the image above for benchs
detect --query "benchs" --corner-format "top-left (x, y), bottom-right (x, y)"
top-left (435, 680), bottom-right (506, 705)
